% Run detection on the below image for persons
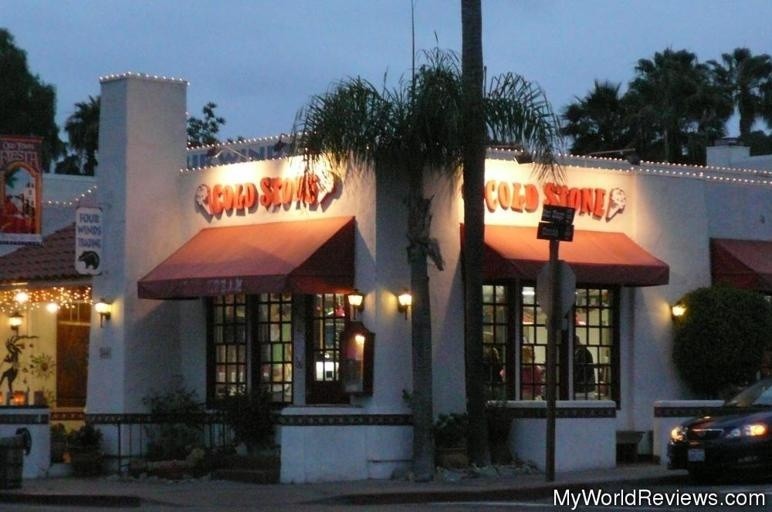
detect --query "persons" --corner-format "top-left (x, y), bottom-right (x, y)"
top-left (487, 347), bottom-right (505, 391)
top-left (520, 343), bottom-right (544, 400)
top-left (573, 335), bottom-right (596, 400)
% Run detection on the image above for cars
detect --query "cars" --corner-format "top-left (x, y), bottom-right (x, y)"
top-left (667, 376), bottom-right (771, 466)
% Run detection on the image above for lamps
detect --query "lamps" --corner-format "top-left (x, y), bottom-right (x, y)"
top-left (346, 288), bottom-right (365, 322)
top-left (397, 286), bottom-right (414, 321)
